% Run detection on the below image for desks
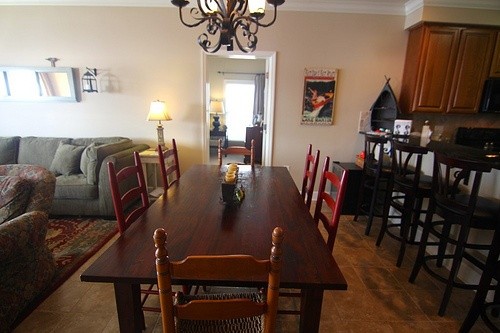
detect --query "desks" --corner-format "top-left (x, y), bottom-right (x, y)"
top-left (80, 165), bottom-right (349, 333)
top-left (210, 128), bottom-right (227, 157)
top-left (139, 148), bottom-right (174, 200)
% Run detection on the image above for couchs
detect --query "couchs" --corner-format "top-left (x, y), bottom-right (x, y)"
top-left (0, 135), bottom-right (139, 218)
top-left (0, 162), bottom-right (56, 333)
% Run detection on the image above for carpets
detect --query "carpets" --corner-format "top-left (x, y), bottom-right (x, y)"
top-left (46, 213), bottom-right (119, 288)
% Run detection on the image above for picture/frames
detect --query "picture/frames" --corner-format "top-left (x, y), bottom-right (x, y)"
top-left (299, 75), bottom-right (337, 125)
top-left (0, 65), bottom-right (78, 103)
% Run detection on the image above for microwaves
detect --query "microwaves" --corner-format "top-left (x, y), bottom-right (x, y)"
top-left (481, 76), bottom-right (500, 112)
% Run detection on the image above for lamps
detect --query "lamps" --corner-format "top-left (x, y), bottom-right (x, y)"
top-left (171, 0), bottom-right (285, 54)
top-left (147, 100), bottom-right (173, 151)
top-left (209, 101), bottom-right (225, 131)
top-left (81, 67), bottom-right (98, 93)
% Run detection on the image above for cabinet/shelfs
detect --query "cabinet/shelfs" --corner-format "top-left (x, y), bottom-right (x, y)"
top-left (398, 23), bottom-right (500, 119)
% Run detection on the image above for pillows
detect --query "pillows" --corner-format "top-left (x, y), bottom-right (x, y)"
top-left (49, 141), bottom-right (102, 176)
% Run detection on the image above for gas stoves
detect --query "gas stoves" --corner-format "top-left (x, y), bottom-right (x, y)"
top-left (455, 126), bottom-right (500, 162)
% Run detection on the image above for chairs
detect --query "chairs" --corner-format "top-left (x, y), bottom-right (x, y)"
top-left (158, 138), bottom-right (181, 192)
top-left (216, 139), bottom-right (256, 165)
top-left (300, 144), bottom-right (321, 210)
top-left (350, 132), bottom-right (500, 333)
top-left (311, 156), bottom-right (349, 252)
top-left (152, 225), bottom-right (284, 333)
top-left (107, 151), bottom-right (149, 235)
